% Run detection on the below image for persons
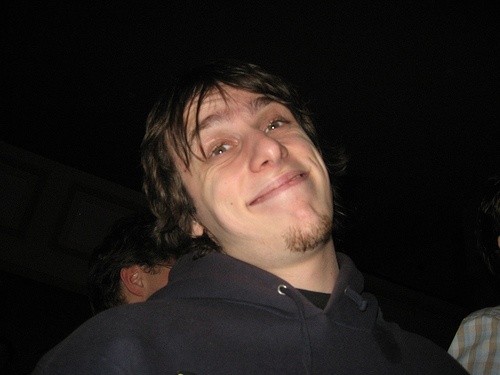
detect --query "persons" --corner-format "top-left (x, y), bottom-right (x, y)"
top-left (29, 48), bottom-right (470, 375)
top-left (449, 182), bottom-right (500, 375)
top-left (85, 215), bottom-right (178, 315)
